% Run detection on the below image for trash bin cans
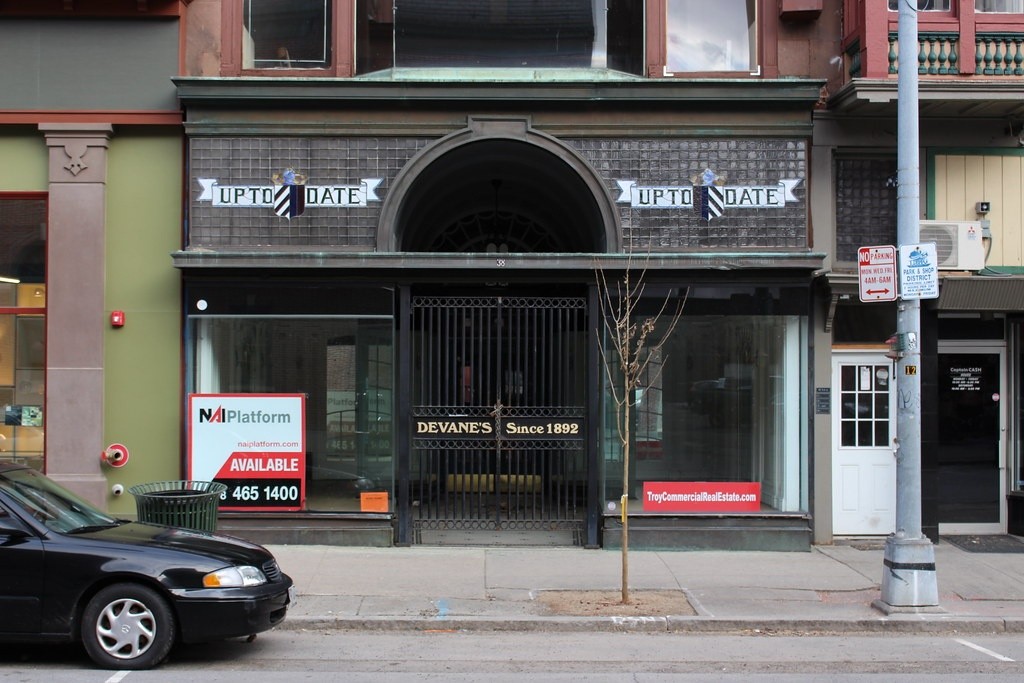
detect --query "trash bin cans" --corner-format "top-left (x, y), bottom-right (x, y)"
top-left (127, 479), bottom-right (229, 532)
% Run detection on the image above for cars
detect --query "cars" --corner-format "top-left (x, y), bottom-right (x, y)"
top-left (0, 457), bottom-right (293, 671)
top-left (688, 366), bottom-right (781, 433)
